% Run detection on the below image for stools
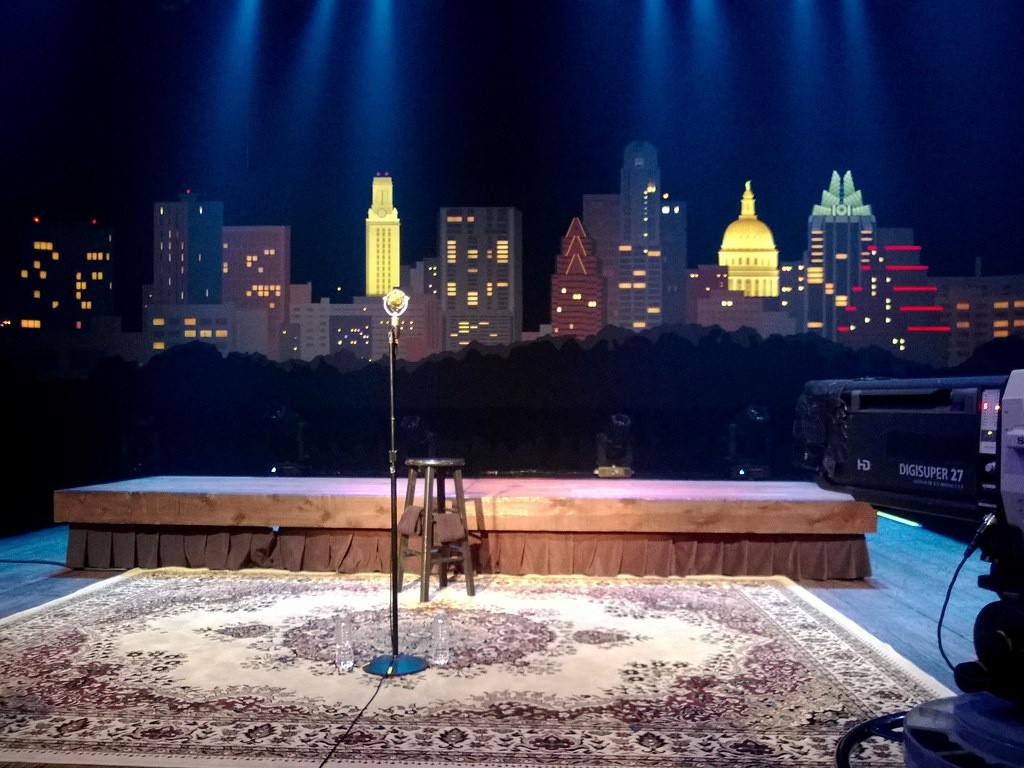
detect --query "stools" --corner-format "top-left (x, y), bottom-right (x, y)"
top-left (397, 457), bottom-right (475, 603)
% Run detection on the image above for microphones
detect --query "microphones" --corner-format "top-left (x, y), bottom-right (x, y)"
top-left (383, 289), bottom-right (410, 344)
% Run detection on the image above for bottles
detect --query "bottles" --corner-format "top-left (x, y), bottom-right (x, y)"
top-left (335, 607), bottom-right (354, 668)
top-left (432, 608), bottom-right (450, 665)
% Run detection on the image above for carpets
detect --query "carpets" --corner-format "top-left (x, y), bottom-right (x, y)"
top-left (0, 566), bottom-right (960, 768)
